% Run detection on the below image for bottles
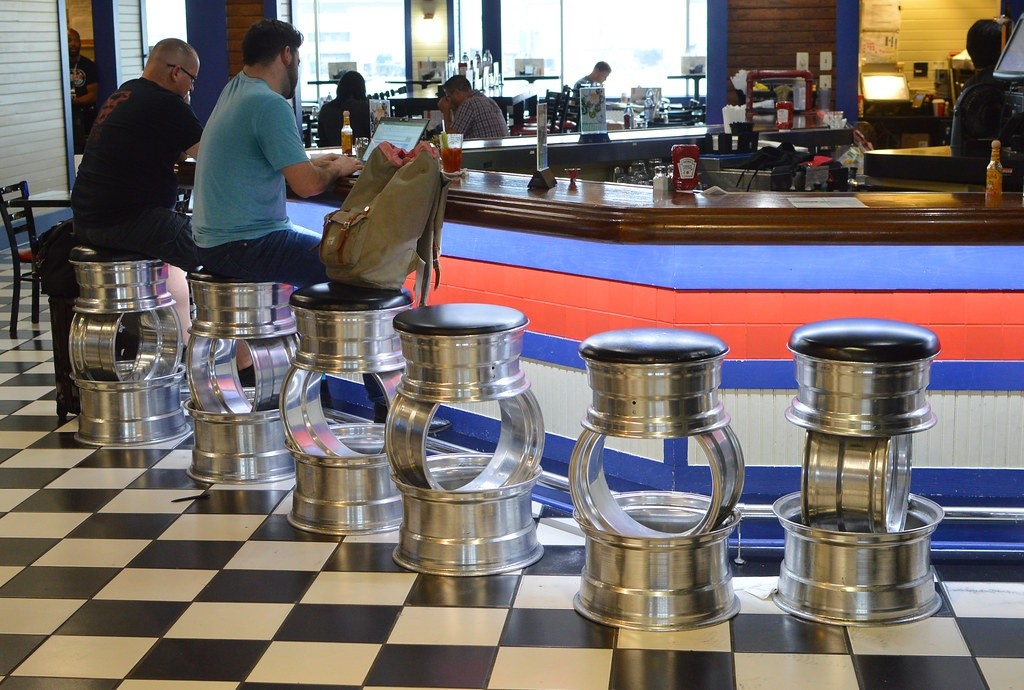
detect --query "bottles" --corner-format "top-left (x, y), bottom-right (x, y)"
top-left (612, 157), bottom-right (674, 191)
top-left (340, 111), bottom-right (353, 157)
top-left (984, 140), bottom-right (1004, 209)
top-left (623, 98), bottom-right (635, 129)
top-left (446, 49), bottom-right (494, 79)
top-left (644, 97), bottom-right (654, 127)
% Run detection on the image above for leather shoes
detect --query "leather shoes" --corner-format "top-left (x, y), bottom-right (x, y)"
top-left (374, 402), bottom-right (453, 433)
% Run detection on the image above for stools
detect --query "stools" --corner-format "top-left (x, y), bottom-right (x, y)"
top-left (769, 319), bottom-right (943, 628)
top-left (569, 327), bottom-right (745, 632)
top-left (71, 244), bottom-right (186, 448)
top-left (388, 303), bottom-right (547, 578)
top-left (183, 268), bottom-right (299, 484)
top-left (278, 280), bottom-right (416, 536)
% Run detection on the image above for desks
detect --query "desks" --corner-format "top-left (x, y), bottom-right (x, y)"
top-left (860, 111), bottom-right (954, 153)
top-left (385, 79), bottom-right (535, 138)
top-left (8, 189), bottom-right (71, 332)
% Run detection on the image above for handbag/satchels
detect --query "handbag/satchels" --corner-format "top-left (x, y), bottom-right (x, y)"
top-left (736, 143), bottom-right (811, 191)
top-left (34, 217), bottom-right (75, 297)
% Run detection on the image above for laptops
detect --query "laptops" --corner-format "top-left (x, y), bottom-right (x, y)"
top-left (347, 117), bottom-right (431, 177)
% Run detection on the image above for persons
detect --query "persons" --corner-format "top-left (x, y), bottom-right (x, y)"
top-left (190, 18), bottom-right (365, 288)
top-left (437, 75), bottom-right (509, 139)
top-left (67, 28), bottom-right (98, 155)
top-left (69, 38), bottom-right (203, 273)
top-left (316, 71), bottom-right (370, 147)
top-left (959, 19), bottom-right (1010, 145)
top-left (570, 61), bottom-right (611, 121)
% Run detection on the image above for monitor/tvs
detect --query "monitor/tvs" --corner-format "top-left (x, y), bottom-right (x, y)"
top-left (860, 71), bottom-right (909, 104)
top-left (992, 14), bottom-right (1024, 86)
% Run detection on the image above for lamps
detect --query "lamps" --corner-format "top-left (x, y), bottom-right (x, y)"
top-left (420, 0), bottom-right (437, 19)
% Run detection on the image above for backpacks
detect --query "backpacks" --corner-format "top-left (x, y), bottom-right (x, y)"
top-left (314, 141), bottom-right (450, 309)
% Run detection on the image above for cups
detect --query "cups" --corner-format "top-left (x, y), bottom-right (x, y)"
top-left (827, 111), bottom-right (843, 129)
top-left (438, 133), bottom-right (464, 187)
top-left (932, 98), bottom-right (945, 118)
top-left (354, 137), bottom-right (368, 160)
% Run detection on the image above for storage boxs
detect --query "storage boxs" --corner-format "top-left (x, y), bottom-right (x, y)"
top-left (899, 132), bottom-right (930, 150)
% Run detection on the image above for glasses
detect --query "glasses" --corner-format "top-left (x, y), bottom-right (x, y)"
top-left (167, 63), bottom-right (198, 83)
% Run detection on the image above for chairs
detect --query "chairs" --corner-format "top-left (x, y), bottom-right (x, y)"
top-left (302, 87), bottom-right (406, 149)
top-left (0, 180), bottom-right (43, 332)
top-left (510, 85), bottom-right (581, 136)
